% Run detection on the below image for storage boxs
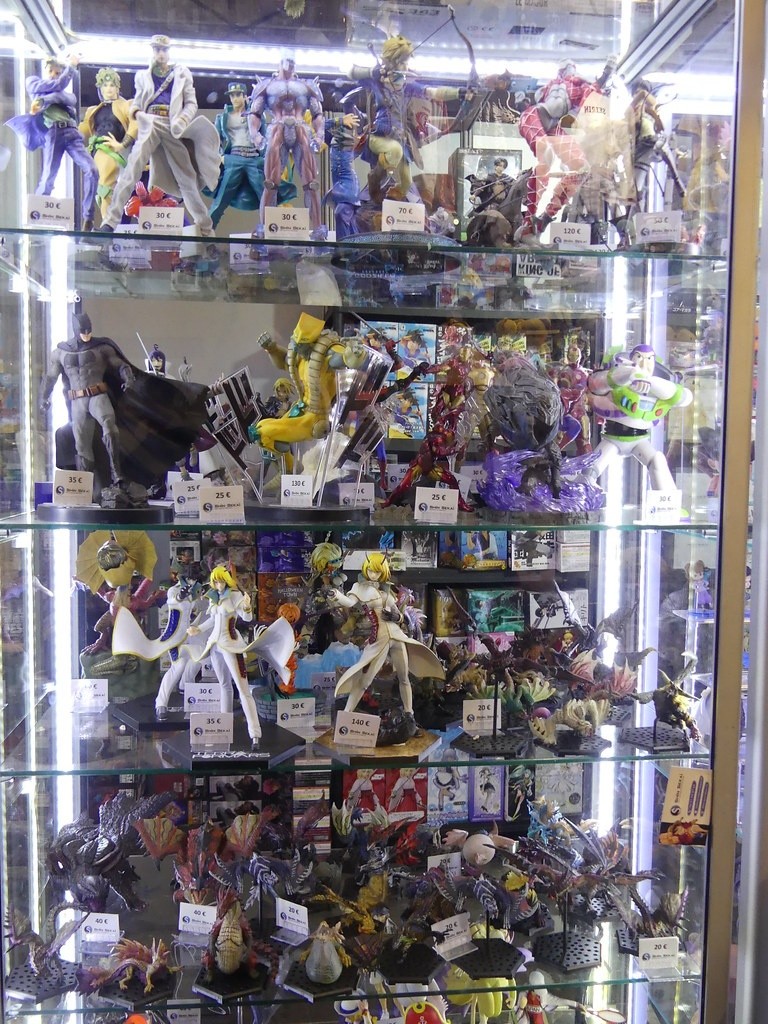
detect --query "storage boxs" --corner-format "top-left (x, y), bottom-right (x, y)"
top-left (157, 122), bottom-right (593, 879)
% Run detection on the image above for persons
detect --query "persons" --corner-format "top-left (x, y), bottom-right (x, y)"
top-left (684, 560), bottom-right (752, 615)
top-left (41, 311), bottom-right (210, 506)
top-left (549, 344), bottom-right (688, 519)
top-left (27, 35), bottom-right (694, 236)
top-left (111, 545), bottom-right (447, 746)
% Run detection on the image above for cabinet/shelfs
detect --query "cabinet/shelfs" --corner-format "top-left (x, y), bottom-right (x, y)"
top-left (0, 0), bottom-right (768, 1024)
